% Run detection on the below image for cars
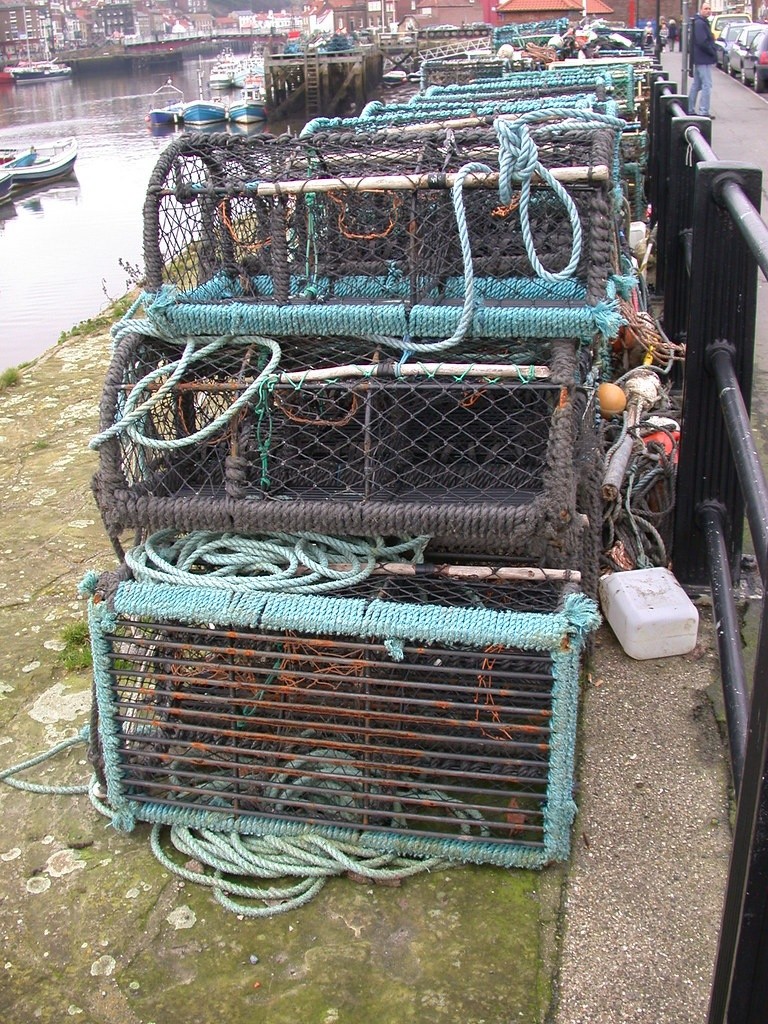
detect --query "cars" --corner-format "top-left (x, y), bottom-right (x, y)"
top-left (740, 28), bottom-right (768, 93)
top-left (727, 24), bottom-right (768, 79)
top-left (712, 22), bottom-right (761, 73)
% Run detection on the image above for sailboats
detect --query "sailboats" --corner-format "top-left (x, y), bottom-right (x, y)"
top-left (2, 5), bottom-right (73, 84)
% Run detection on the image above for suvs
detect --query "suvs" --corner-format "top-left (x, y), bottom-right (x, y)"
top-left (711, 14), bottom-right (754, 41)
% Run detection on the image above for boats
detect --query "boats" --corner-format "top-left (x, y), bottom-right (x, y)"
top-left (0, 165), bottom-right (16, 201)
top-left (207, 27), bottom-right (354, 91)
top-left (0, 134), bottom-right (81, 183)
top-left (225, 84), bottom-right (268, 124)
top-left (381, 70), bottom-right (407, 86)
top-left (145, 74), bottom-right (190, 126)
top-left (0, 145), bottom-right (39, 168)
top-left (405, 69), bottom-right (421, 84)
top-left (181, 54), bottom-right (230, 126)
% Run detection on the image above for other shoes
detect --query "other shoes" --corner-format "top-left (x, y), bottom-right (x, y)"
top-left (703, 115), bottom-right (715, 119)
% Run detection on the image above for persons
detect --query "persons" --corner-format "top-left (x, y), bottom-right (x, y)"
top-left (688, 2), bottom-right (718, 120)
top-left (644, 16), bottom-right (677, 53)
